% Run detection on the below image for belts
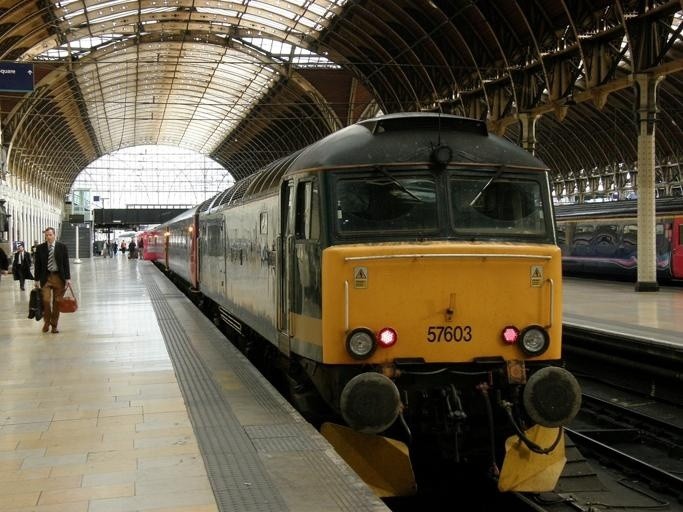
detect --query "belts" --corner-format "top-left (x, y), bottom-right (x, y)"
top-left (48, 271), bottom-right (58, 275)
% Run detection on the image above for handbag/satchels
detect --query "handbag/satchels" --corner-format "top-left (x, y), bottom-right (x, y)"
top-left (28, 288), bottom-right (43, 320)
top-left (60, 296), bottom-right (77, 312)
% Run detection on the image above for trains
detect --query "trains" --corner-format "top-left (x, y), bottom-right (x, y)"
top-left (137, 112), bottom-right (583, 499)
top-left (553, 197), bottom-right (683, 287)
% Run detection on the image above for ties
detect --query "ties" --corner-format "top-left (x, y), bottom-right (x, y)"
top-left (47, 245), bottom-right (54, 270)
top-left (21, 253), bottom-right (23, 266)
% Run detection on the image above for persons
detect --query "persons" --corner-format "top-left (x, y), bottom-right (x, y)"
top-left (121, 239), bottom-right (128, 257)
top-left (12, 245), bottom-right (36, 292)
top-left (31, 241), bottom-right (40, 266)
top-left (138, 237), bottom-right (145, 261)
top-left (129, 239), bottom-right (137, 260)
top-left (103, 240), bottom-right (117, 259)
top-left (33, 228), bottom-right (72, 334)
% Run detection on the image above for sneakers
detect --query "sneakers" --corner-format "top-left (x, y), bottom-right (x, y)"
top-left (43, 322), bottom-right (58, 333)
